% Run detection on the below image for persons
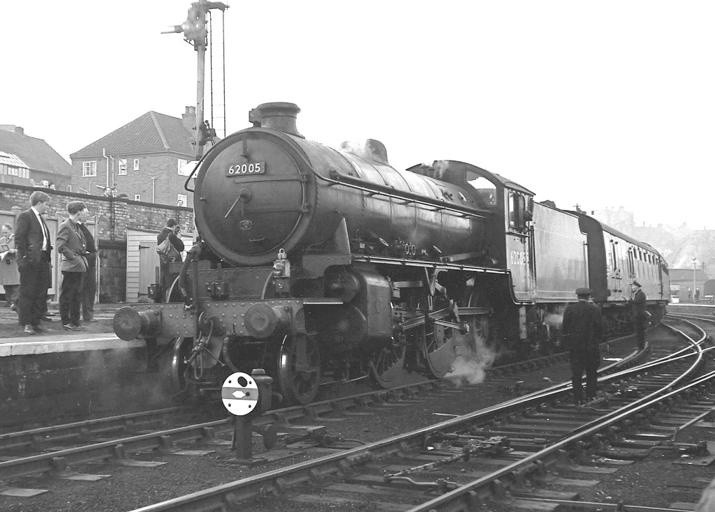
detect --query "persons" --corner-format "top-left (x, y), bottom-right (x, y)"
top-left (0, 223), bottom-right (22, 311)
top-left (563, 288), bottom-right (606, 405)
top-left (157, 219), bottom-right (185, 290)
top-left (16, 191), bottom-right (53, 335)
top-left (55, 201), bottom-right (89, 331)
top-left (75, 204), bottom-right (99, 323)
top-left (627, 280), bottom-right (646, 350)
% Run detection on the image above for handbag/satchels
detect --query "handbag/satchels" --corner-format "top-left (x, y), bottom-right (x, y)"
top-left (156, 240), bottom-right (178, 262)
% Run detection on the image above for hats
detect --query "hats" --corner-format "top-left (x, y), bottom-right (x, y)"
top-left (632, 281), bottom-right (642, 287)
top-left (576, 287), bottom-right (590, 297)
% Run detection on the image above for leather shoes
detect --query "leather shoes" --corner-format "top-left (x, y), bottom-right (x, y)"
top-left (24, 313), bottom-right (97, 335)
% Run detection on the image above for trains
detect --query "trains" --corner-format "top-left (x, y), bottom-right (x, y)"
top-left (111, 101), bottom-right (672, 408)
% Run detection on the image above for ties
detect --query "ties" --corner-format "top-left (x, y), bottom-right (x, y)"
top-left (39, 214), bottom-right (48, 240)
